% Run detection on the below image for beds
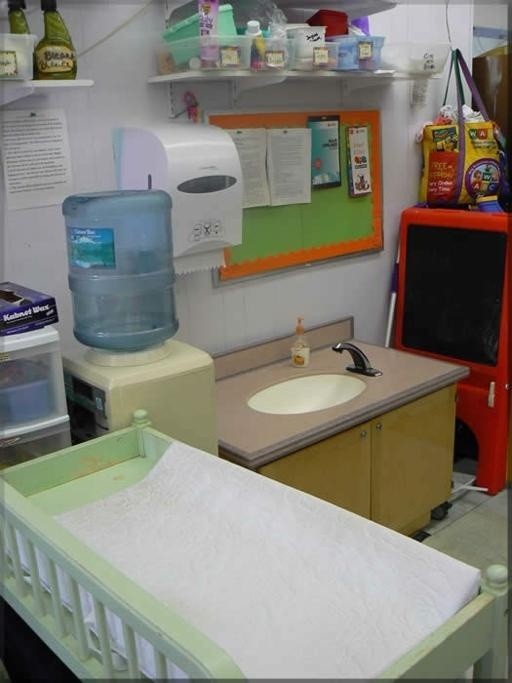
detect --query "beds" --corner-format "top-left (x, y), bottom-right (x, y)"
top-left (1, 406), bottom-right (507, 678)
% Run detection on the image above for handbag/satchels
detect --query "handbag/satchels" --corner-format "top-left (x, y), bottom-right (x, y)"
top-left (417, 48), bottom-right (505, 207)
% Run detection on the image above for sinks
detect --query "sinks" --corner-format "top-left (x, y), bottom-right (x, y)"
top-left (248, 372), bottom-right (367, 414)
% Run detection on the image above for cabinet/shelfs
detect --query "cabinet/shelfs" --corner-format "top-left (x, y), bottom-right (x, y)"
top-left (258, 384), bottom-right (457, 536)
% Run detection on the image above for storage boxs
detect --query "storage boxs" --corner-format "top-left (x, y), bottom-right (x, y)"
top-left (0, 326), bottom-right (72, 469)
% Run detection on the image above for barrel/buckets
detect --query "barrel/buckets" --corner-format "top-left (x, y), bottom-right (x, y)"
top-left (62, 190), bottom-right (179, 353)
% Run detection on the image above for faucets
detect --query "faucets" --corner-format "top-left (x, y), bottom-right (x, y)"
top-left (331, 340), bottom-right (383, 377)
